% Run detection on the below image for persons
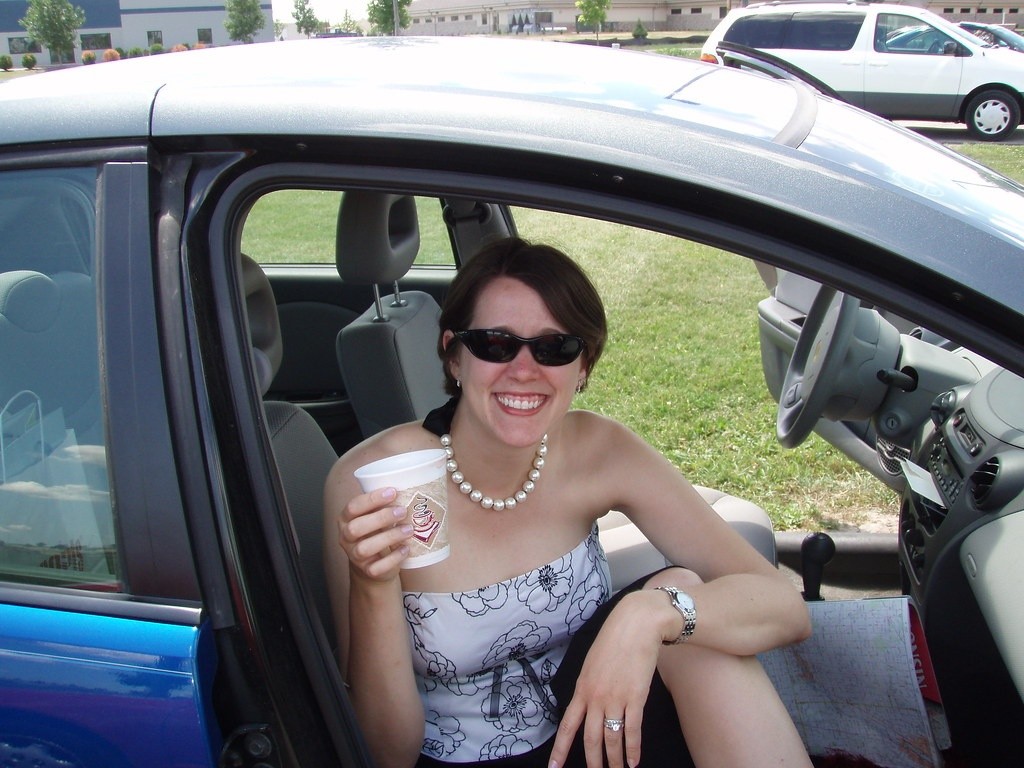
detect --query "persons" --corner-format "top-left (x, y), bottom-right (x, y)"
top-left (323, 237), bottom-right (816, 768)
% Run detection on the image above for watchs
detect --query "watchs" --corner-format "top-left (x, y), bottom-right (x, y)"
top-left (654, 586), bottom-right (698, 646)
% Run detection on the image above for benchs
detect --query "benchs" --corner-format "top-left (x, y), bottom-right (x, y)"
top-left (0, 266), bottom-right (117, 579)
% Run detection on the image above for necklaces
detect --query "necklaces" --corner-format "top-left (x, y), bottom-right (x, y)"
top-left (439, 432), bottom-right (549, 512)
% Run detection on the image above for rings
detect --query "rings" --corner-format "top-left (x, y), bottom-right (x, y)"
top-left (604, 719), bottom-right (624, 731)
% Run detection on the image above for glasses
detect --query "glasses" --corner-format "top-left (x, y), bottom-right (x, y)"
top-left (454, 330), bottom-right (588, 367)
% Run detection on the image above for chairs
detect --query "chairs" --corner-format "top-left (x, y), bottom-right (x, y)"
top-left (235, 251), bottom-right (340, 653)
top-left (336, 189), bottom-right (780, 590)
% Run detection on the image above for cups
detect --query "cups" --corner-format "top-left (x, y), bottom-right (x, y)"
top-left (353, 448), bottom-right (452, 570)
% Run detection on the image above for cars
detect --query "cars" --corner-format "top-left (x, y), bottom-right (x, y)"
top-left (698, 1), bottom-right (1024, 141)
top-left (0, 34), bottom-right (1024, 768)
top-left (886, 20), bottom-right (1024, 57)
